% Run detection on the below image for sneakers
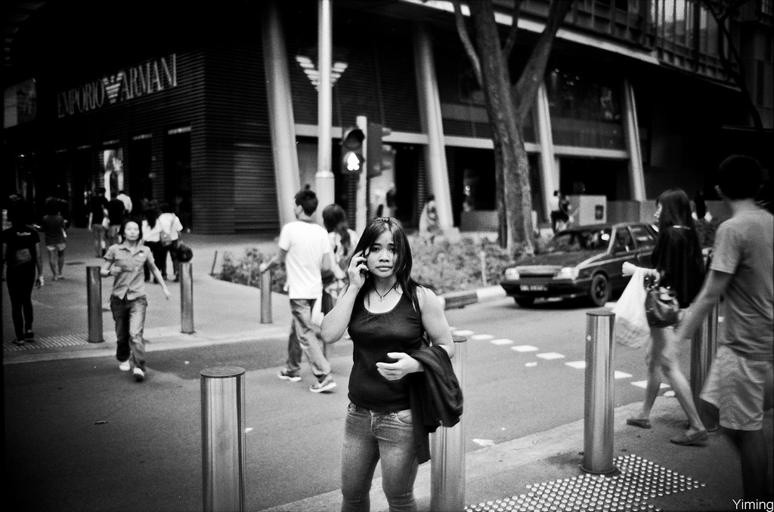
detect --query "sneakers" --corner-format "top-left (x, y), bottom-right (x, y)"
top-left (133, 366), bottom-right (144, 378)
top-left (13, 336), bottom-right (25, 344)
top-left (626, 416), bottom-right (651, 429)
top-left (671, 429), bottom-right (707, 445)
top-left (145, 271), bottom-right (179, 283)
top-left (25, 332), bottom-right (34, 342)
top-left (119, 360), bottom-right (130, 372)
top-left (310, 375), bottom-right (335, 393)
top-left (280, 369), bottom-right (301, 381)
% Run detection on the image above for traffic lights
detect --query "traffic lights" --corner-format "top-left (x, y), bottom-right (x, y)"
top-left (340, 123), bottom-right (365, 174)
top-left (369, 125), bottom-right (393, 177)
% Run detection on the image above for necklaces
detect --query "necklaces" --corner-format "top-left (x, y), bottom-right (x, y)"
top-left (374, 285), bottom-right (394, 303)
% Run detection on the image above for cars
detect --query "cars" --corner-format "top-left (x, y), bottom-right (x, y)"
top-left (501, 223), bottom-right (664, 309)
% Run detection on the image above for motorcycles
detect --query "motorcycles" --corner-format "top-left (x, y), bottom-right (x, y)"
top-left (547, 181), bottom-right (587, 233)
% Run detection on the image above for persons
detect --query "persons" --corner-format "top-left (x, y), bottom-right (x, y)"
top-left (660, 155), bottom-right (774, 512)
top-left (2, 181), bottom-right (183, 380)
top-left (690, 191), bottom-right (713, 222)
top-left (322, 217), bottom-right (455, 512)
top-left (550, 191), bottom-right (571, 235)
top-left (322, 204), bottom-right (360, 359)
top-left (622, 188), bottom-right (709, 444)
top-left (259, 183), bottom-right (336, 393)
top-left (694, 202), bottom-right (714, 249)
top-left (419, 194), bottom-right (436, 245)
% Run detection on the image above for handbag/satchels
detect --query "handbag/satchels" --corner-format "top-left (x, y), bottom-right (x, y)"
top-left (160, 230), bottom-right (172, 247)
top-left (643, 268), bottom-right (679, 327)
top-left (178, 245), bottom-right (192, 263)
